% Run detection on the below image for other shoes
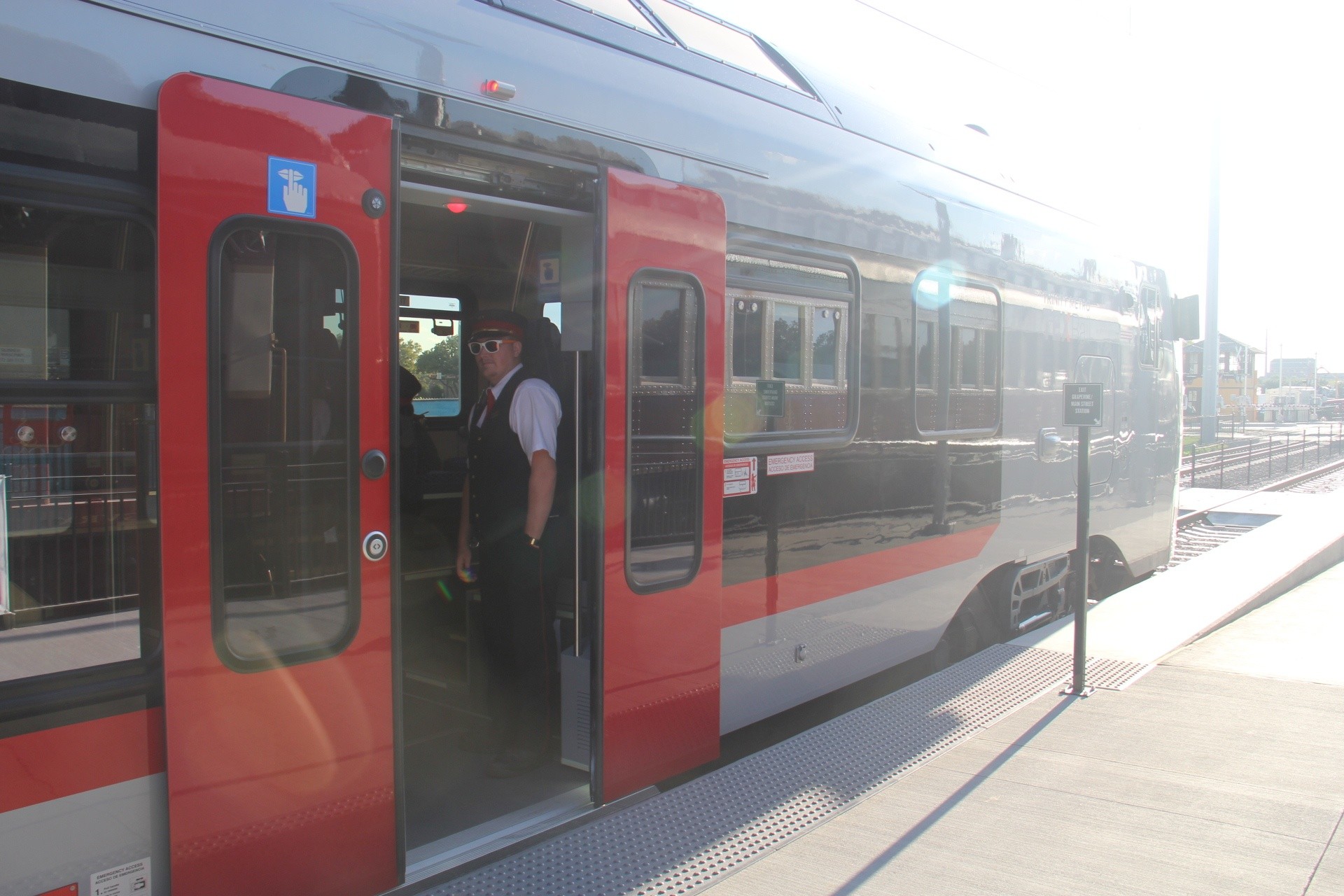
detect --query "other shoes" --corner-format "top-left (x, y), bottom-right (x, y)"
top-left (486, 748), bottom-right (548, 780)
top-left (456, 730), bottom-right (515, 754)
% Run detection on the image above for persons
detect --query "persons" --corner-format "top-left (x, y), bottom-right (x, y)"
top-left (455, 309), bottom-right (564, 745)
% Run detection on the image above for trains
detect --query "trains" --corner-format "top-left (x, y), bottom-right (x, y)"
top-left (0, 0), bottom-right (1212, 896)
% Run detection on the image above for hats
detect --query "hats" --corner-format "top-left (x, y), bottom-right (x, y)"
top-left (467, 308), bottom-right (527, 343)
top-left (399, 365), bottom-right (422, 399)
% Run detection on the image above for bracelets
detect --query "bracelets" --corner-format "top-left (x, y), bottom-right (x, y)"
top-left (521, 533), bottom-right (541, 547)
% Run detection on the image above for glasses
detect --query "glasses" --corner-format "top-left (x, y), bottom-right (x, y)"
top-left (467, 336), bottom-right (517, 356)
